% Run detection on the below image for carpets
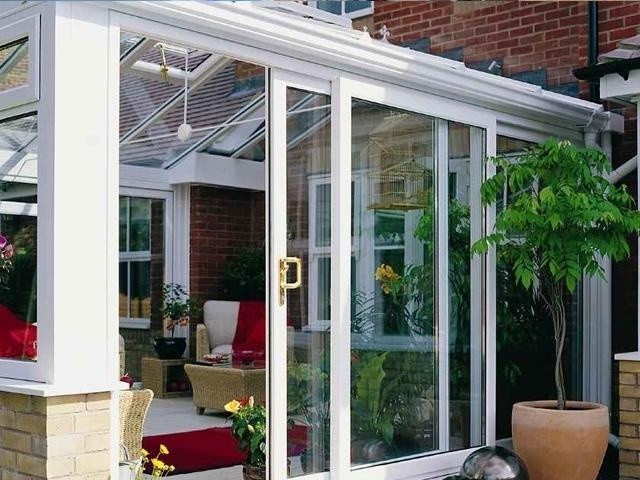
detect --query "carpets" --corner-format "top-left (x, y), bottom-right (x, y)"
top-left (141, 420), bottom-right (309, 477)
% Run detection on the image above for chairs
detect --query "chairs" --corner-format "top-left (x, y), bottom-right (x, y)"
top-left (193, 296), bottom-right (293, 360)
top-left (120, 386), bottom-right (152, 464)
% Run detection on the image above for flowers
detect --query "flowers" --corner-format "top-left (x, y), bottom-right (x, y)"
top-left (220, 394), bottom-right (273, 465)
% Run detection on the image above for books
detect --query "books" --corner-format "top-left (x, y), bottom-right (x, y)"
top-left (191, 360), bottom-right (232, 366)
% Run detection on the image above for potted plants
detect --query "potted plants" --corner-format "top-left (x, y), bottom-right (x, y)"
top-left (340, 289), bottom-right (429, 464)
top-left (470, 137), bottom-right (640, 480)
top-left (152, 277), bottom-right (198, 359)
top-left (393, 187), bottom-right (536, 453)
top-left (286, 361), bottom-right (332, 474)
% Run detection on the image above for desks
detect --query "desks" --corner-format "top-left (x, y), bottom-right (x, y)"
top-left (184, 361), bottom-right (265, 417)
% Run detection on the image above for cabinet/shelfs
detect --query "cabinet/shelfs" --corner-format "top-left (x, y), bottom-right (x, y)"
top-left (141, 357), bottom-right (194, 400)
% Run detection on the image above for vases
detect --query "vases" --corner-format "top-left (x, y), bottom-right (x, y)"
top-left (238, 460), bottom-right (292, 480)
